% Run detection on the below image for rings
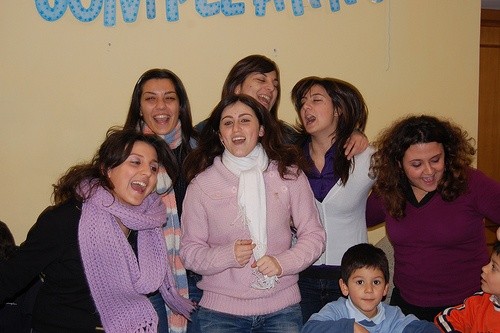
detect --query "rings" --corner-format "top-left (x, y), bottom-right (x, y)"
top-left (240, 240), bottom-right (242, 245)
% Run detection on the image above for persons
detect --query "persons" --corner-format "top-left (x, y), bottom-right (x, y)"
top-left (366, 117), bottom-right (500, 324)
top-left (301, 244), bottom-right (442, 333)
top-left (122, 69), bottom-right (213, 333)
top-left (433, 238), bottom-right (500, 333)
top-left (186, 54), bottom-right (368, 189)
top-left (0, 132), bottom-right (161, 333)
top-left (289, 75), bottom-right (381, 323)
top-left (181, 94), bottom-right (327, 333)
